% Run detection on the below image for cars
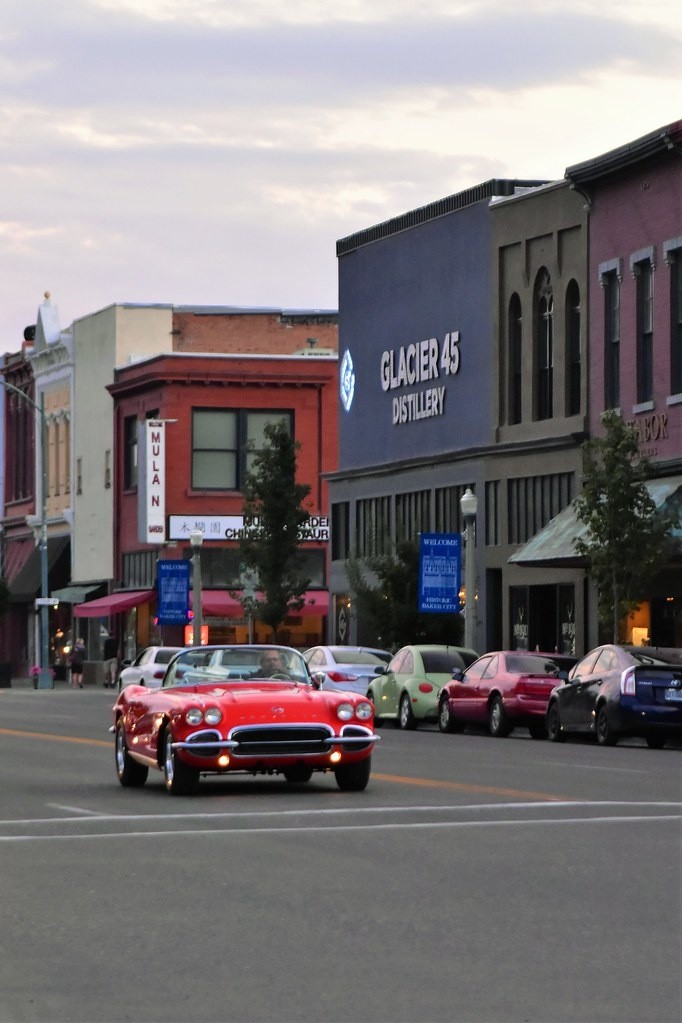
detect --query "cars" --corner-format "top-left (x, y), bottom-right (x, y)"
top-left (182, 646), bottom-right (292, 684)
top-left (544, 643), bottom-right (682, 750)
top-left (436, 650), bottom-right (581, 739)
top-left (366, 645), bottom-right (487, 730)
top-left (116, 645), bottom-right (197, 696)
top-left (287, 645), bottom-right (396, 699)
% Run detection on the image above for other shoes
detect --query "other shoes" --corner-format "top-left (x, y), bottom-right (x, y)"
top-left (79, 683), bottom-right (83, 688)
top-left (103, 683), bottom-right (108, 688)
top-left (111, 683), bottom-right (115, 688)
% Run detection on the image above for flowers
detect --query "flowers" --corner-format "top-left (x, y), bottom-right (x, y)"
top-left (28, 666), bottom-right (56, 679)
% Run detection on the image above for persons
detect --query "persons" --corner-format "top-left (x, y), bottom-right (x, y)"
top-left (535, 643), bottom-right (545, 652)
top-left (246, 649), bottom-right (293, 681)
top-left (69, 646), bottom-right (85, 689)
top-left (103, 632), bottom-right (119, 689)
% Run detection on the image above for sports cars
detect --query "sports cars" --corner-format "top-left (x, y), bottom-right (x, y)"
top-left (108, 644), bottom-right (383, 796)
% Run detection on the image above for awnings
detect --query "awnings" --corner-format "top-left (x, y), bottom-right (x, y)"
top-left (72, 592), bottom-right (154, 618)
top-left (187, 589), bottom-right (328, 617)
top-left (51, 584), bottom-right (103, 603)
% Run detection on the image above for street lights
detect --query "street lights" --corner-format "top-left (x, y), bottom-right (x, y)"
top-left (459, 486), bottom-right (479, 655)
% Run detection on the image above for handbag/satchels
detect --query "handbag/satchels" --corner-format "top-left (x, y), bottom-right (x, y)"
top-left (69, 650), bottom-right (77, 660)
